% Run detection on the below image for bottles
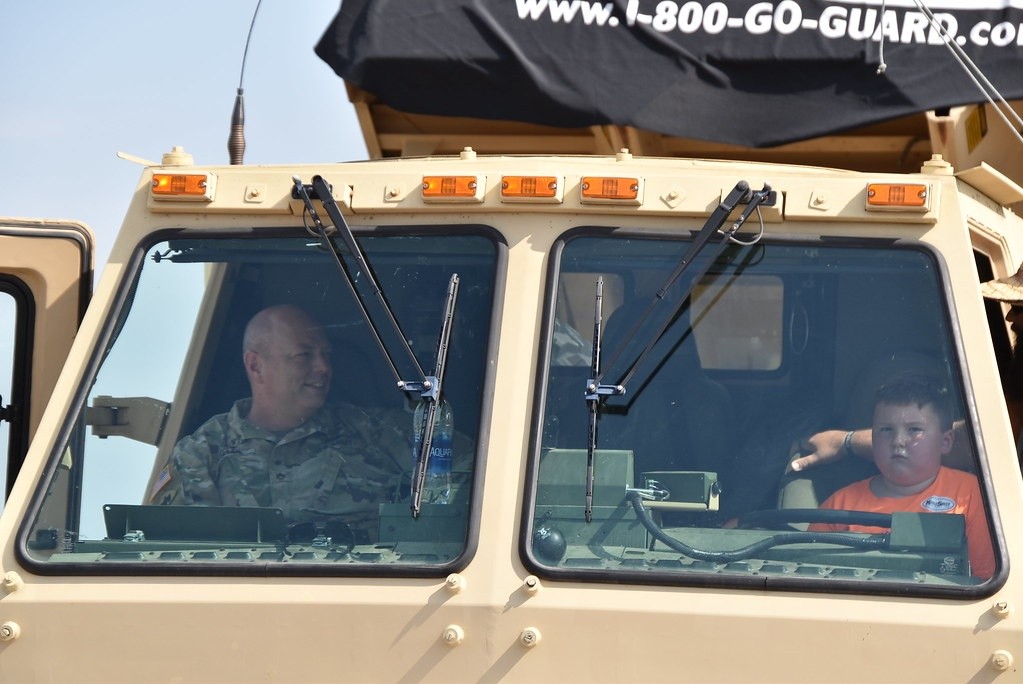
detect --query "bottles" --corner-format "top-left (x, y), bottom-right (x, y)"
top-left (410, 397), bottom-right (453, 505)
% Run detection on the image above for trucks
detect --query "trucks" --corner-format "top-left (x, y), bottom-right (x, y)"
top-left (2, 0), bottom-right (1023, 684)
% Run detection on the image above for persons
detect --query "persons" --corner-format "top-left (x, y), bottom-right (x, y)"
top-left (785, 260), bottom-right (1023, 475)
top-left (154, 303), bottom-right (405, 544)
top-left (806, 371), bottom-right (996, 579)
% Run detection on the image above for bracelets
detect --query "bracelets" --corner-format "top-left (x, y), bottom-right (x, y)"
top-left (845, 430), bottom-right (853, 453)
